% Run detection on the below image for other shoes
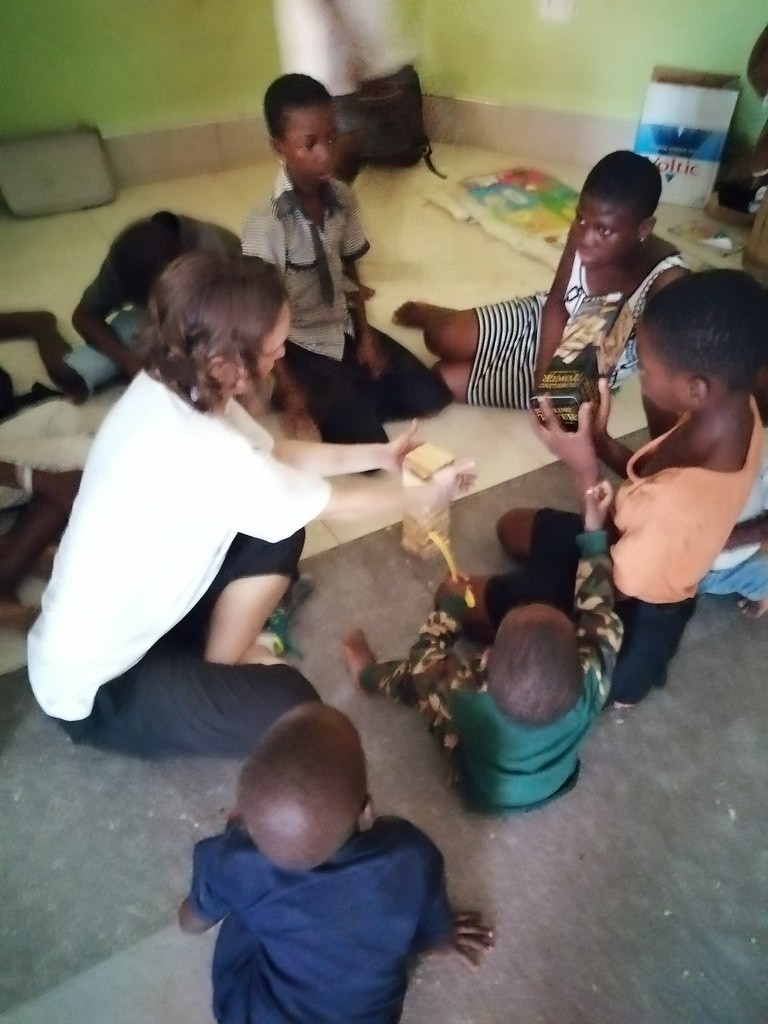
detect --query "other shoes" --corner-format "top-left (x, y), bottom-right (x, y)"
top-left (262, 572), bottom-right (316, 659)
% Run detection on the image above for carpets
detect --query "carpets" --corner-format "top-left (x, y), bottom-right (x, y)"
top-left (1, 428), bottom-right (768, 1023)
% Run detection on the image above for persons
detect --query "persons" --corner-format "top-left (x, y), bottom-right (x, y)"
top-left (239, 74), bottom-right (452, 445)
top-left (390, 152), bottom-right (691, 412)
top-left (344, 479), bottom-right (625, 813)
top-left (434, 269), bottom-right (768, 708)
top-left (1, 210), bottom-right (243, 631)
top-left (179, 705), bottom-right (492, 1024)
top-left (26, 252), bottom-right (426, 760)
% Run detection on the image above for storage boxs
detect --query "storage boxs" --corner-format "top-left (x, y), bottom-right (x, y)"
top-left (633, 66), bottom-right (742, 208)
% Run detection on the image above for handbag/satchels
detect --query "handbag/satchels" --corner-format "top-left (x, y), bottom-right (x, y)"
top-left (358, 66), bottom-right (448, 179)
